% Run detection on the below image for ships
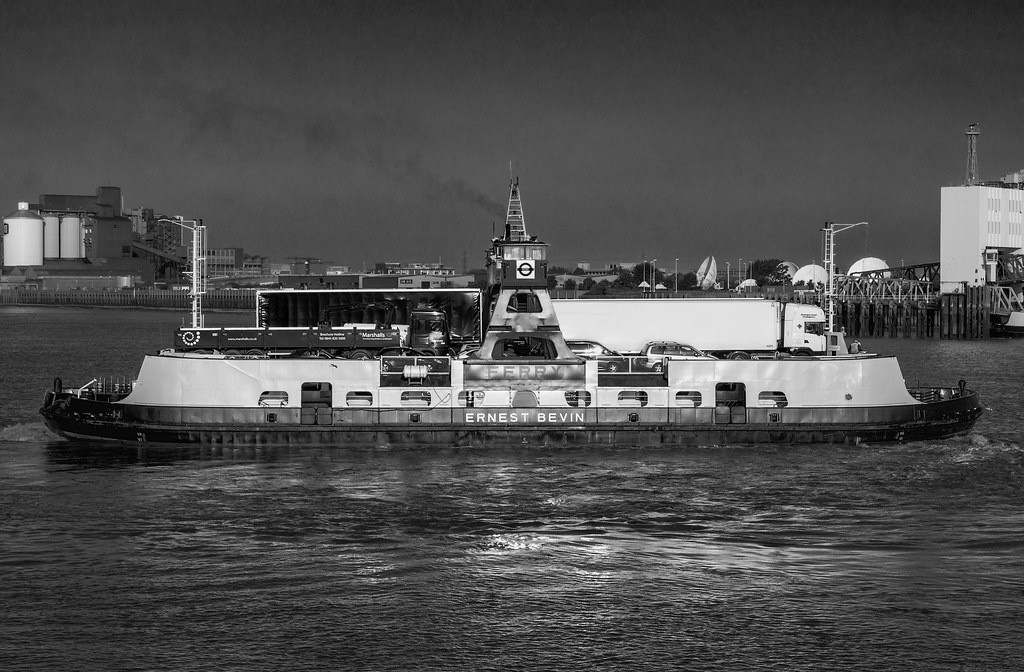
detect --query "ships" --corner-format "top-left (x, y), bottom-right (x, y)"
top-left (38, 158), bottom-right (987, 451)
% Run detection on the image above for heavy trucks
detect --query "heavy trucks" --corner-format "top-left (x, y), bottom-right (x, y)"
top-left (550, 296), bottom-right (828, 357)
top-left (174, 305), bottom-right (452, 358)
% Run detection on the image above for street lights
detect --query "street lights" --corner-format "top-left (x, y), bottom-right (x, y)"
top-left (743, 263), bottom-right (746, 292)
top-left (643, 259), bottom-right (648, 293)
top-left (675, 258), bottom-right (680, 293)
top-left (649, 261), bottom-right (653, 292)
top-left (653, 258), bottom-right (658, 292)
top-left (726, 261), bottom-right (729, 289)
top-left (748, 260), bottom-right (752, 292)
top-left (738, 258), bottom-right (742, 293)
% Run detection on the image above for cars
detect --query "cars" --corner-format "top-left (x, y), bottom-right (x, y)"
top-left (637, 340), bottom-right (720, 373)
top-left (562, 338), bottom-right (626, 373)
top-left (374, 345), bottom-right (446, 375)
